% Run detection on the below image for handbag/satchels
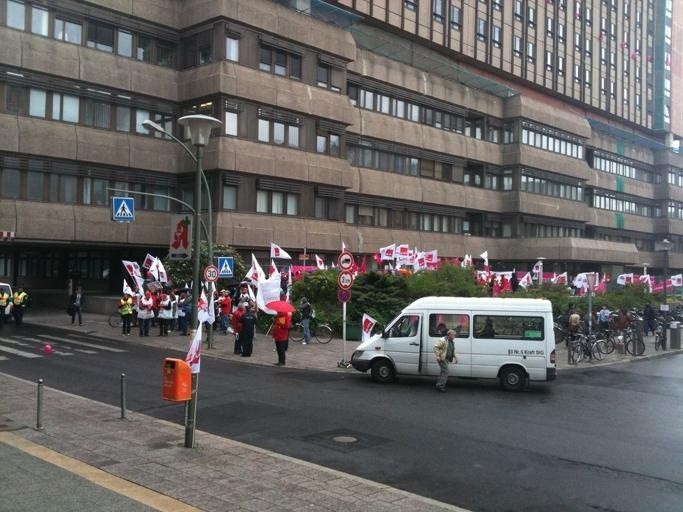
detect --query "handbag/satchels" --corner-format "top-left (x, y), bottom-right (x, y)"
top-left (309, 303), bottom-right (316, 319)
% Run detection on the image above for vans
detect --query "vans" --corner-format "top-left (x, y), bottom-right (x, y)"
top-left (349, 296), bottom-right (556, 391)
top-left (0, 282), bottom-right (13, 318)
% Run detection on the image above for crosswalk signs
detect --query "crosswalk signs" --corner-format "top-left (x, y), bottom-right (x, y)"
top-left (218, 256), bottom-right (233, 278)
top-left (111, 196), bottom-right (134, 221)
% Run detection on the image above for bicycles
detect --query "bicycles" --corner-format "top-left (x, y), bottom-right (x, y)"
top-left (551, 305), bottom-right (682, 365)
top-left (288, 312), bottom-right (333, 345)
top-left (107, 304), bottom-right (136, 328)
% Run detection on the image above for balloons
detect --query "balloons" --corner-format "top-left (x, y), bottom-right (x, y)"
top-left (43, 344), bottom-right (52, 353)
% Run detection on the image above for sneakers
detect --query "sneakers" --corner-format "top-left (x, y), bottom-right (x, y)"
top-left (436, 384), bottom-right (447, 392)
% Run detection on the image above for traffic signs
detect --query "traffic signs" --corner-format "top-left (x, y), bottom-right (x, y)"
top-left (203, 266), bottom-right (219, 283)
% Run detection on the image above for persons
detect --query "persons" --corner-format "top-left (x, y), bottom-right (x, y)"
top-left (437, 324), bottom-right (450, 334)
top-left (113, 286), bottom-right (313, 357)
top-left (560, 299), bottom-right (656, 353)
top-left (69, 286), bottom-right (84, 326)
top-left (11, 285), bottom-right (28, 329)
top-left (432, 329), bottom-right (459, 392)
top-left (0, 287), bottom-right (10, 329)
top-left (270, 311), bottom-right (291, 367)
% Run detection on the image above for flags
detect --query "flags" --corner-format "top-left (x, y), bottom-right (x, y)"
top-left (242, 243), bottom-right (293, 315)
top-left (314, 254), bottom-right (327, 272)
top-left (360, 313), bottom-right (378, 343)
top-left (329, 240), bottom-right (683, 299)
top-left (121, 253), bottom-right (167, 296)
top-left (182, 320), bottom-right (201, 374)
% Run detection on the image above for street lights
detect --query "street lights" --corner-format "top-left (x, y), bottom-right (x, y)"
top-left (141, 119), bottom-right (214, 350)
top-left (176, 111), bottom-right (224, 449)
top-left (659, 238), bottom-right (670, 351)
top-left (535, 257), bottom-right (548, 288)
top-left (640, 262), bottom-right (649, 277)
